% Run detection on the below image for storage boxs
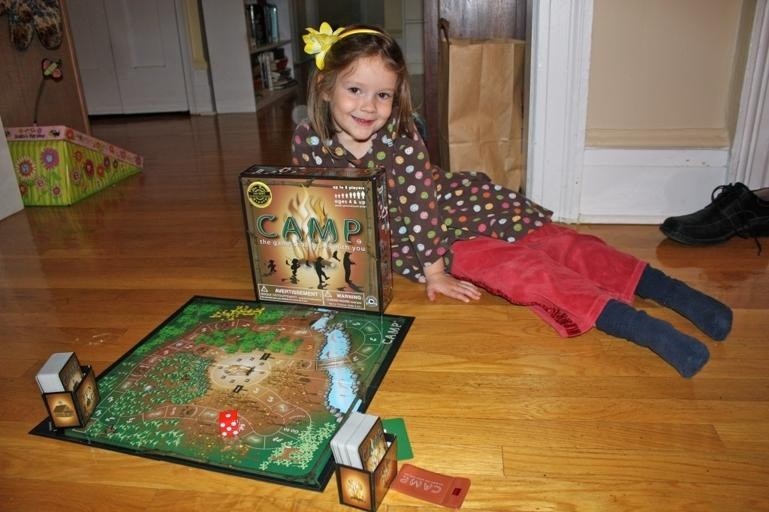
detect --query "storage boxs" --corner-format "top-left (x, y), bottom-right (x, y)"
top-left (239, 165), bottom-right (394, 315)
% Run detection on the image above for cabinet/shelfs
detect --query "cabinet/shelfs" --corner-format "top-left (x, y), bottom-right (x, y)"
top-left (199, 0), bottom-right (299, 113)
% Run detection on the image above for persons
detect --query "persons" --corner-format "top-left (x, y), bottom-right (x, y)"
top-left (290, 22), bottom-right (735, 381)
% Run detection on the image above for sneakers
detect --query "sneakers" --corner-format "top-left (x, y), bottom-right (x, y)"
top-left (659, 182), bottom-right (768, 256)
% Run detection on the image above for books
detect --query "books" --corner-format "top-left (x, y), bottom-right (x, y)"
top-left (244, 0), bottom-right (299, 104)
